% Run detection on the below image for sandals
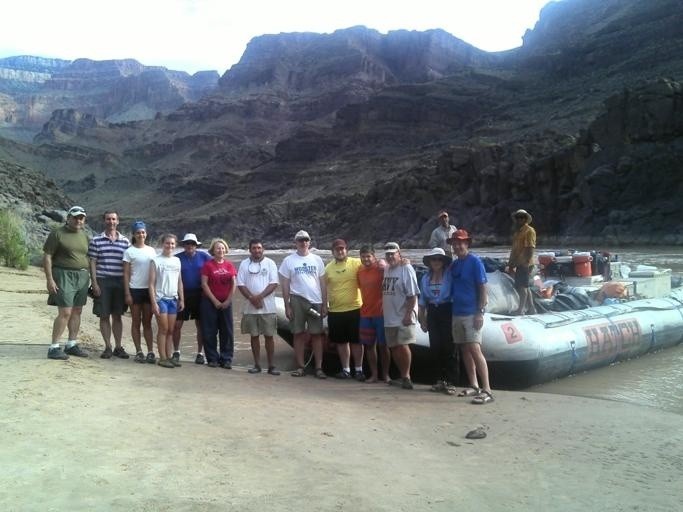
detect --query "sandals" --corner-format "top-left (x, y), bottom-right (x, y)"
top-left (431, 379), bottom-right (495, 403)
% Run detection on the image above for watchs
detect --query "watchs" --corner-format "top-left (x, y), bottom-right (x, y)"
top-left (476, 307), bottom-right (486, 315)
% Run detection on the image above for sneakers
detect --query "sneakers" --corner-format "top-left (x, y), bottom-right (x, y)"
top-left (113, 347), bottom-right (129, 358)
top-left (136, 351), bottom-right (182, 367)
top-left (65, 345), bottom-right (88, 357)
top-left (196, 352), bottom-right (232, 369)
top-left (248, 366), bottom-right (261, 373)
top-left (314, 368), bottom-right (328, 378)
top-left (335, 370), bottom-right (392, 384)
top-left (267, 367), bottom-right (280, 375)
top-left (48, 347), bottom-right (68, 359)
top-left (402, 376), bottom-right (413, 389)
top-left (292, 367), bottom-right (305, 376)
top-left (101, 347), bottom-right (112, 358)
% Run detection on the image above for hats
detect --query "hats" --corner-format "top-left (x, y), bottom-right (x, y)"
top-left (132, 222), bottom-right (145, 230)
top-left (512, 209), bottom-right (532, 224)
top-left (68, 206), bottom-right (86, 217)
top-left (384, 242), bottom-right (399, 254)
top-left (181, 233), bottom-right (202, 246)
top-left (446, 230), bottom-right (471, 244)
top-left (294, 231), bottom-right (311, 241)
top-left (332, 240), bottom-right (345, 249)
top-left (423, 248), bottom-right (452, 267)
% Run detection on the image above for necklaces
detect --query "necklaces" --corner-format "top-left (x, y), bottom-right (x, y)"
top-left (247, 254), bottom-right (262, 275)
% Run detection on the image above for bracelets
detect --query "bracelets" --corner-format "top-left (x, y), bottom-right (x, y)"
top-left (248, 295), bottom-right (253, 300)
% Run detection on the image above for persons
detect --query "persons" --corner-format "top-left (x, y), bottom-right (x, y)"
top-left (430, 210), bottom-right (458, 266)
top-left (506, 208), bottom-right (537, 316)
top-left (198, 238), bottom-right (238, 369)
top-left (278, 229), bottom-right (329, 379)
top-left (325, 238), bottom-right (385, 382)
top-left (357, 245), bottom-right (410, 385)
top-left (42, 205), bottom-right (89, 360)
top-left (418, 248), bottom-right (459, 395)
top-left (446, 229), bottom-right (495, 405)
top-left (87, 210), bottom-right (131, 359)
top-left (236, 238), bottom-right (281, 375)
top-left (380, 241), bottom-right (422, 390)
top-left (173, 233), bottom-right (213, 364)
top-left (148, 233), bottom-right (185, 368)
top-left (122, 221), bottom-right (157, 362)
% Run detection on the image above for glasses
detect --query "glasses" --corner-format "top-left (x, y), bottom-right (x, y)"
top-left (248, 265), bottom-right (261, 274)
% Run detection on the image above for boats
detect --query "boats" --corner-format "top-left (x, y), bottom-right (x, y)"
top-left (268, 249), bottom-right (683, 393)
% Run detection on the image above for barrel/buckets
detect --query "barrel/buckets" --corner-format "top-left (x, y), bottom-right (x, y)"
top-left (538, 251), bottom-right (593, 277)
